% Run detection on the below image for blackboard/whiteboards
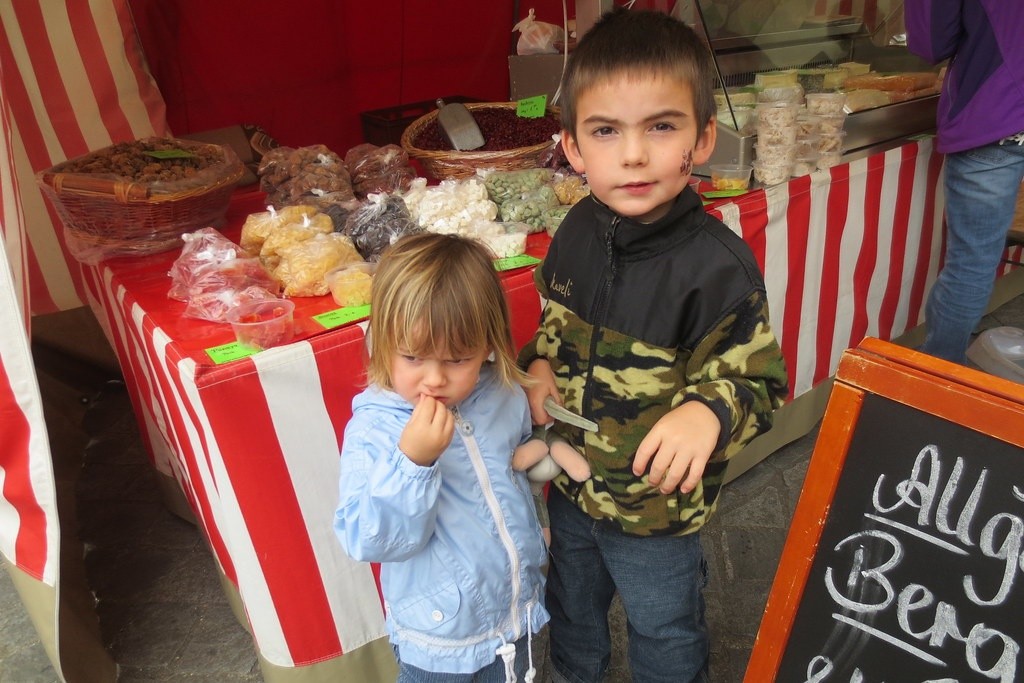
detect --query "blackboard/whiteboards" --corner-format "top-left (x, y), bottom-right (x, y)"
top-left (736, 334), bottom-right (1024, 683)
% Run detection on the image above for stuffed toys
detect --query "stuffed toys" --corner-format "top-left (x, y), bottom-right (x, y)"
top-left (508, 425), bottom-right (592, 546)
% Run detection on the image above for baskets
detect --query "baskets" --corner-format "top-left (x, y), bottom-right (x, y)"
top-left (34, 138), bottom-right (245, 256)
top-left (401, 101), bottom-right (562, 181)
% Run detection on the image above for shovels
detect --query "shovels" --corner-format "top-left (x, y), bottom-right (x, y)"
top-left (435, 97), bottom-right (486, 152)
top-left (963, 326), bottom-right (1024, 384)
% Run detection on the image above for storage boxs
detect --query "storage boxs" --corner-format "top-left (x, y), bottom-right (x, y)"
top-left (360, 95), bottom-right (489, 148)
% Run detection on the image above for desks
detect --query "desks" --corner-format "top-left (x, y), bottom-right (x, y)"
top-left (64, 125), bottom-right (1024, 683)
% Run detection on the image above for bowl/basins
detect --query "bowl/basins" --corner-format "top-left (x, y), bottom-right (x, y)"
top-left (541, 205), bottom-right (574, 239)
top-left (475, 222), bottom-right (530, 257)
top-left (228, 299), bottom-right (295, 349)
top-left (688, 176), bottom-right (701, 194)
top-left (709, 86), bottom-right (847, 191)
top-left (324, 262), bottom-right (377, 306)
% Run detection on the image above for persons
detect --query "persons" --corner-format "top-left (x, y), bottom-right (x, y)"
top-left (514, 10), bottom-right (788, 683)
top-left (335, 233), bottom-right (548, 683)
top-left (904, 0), bottom-right (1024, 368)
